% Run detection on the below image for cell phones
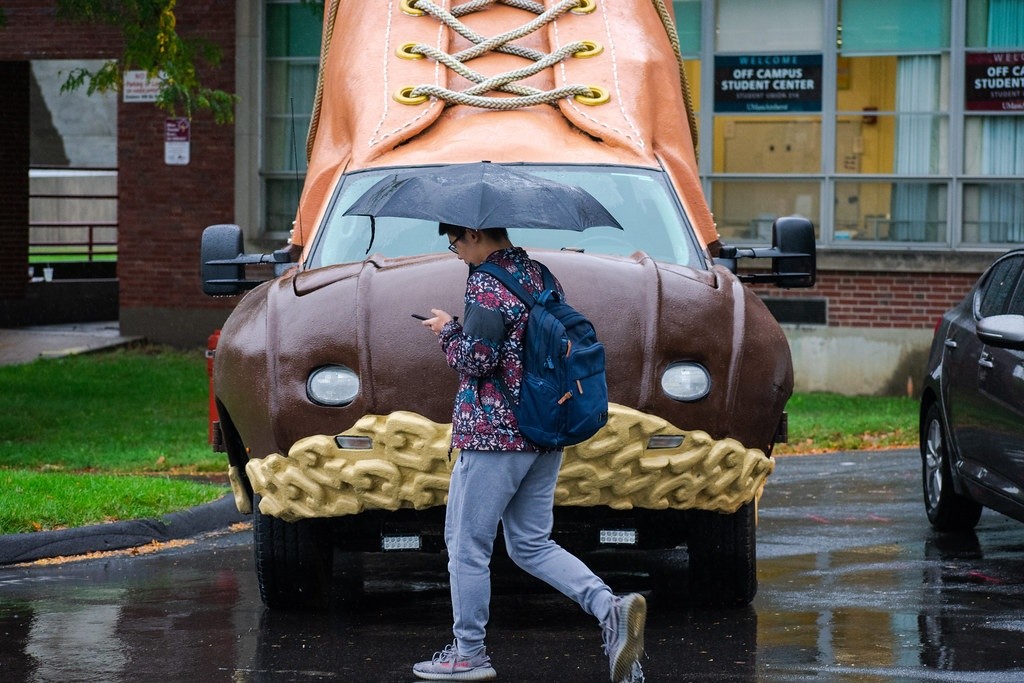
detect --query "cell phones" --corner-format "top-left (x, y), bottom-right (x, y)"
top-left (411, 314), bottom-right (429, 321)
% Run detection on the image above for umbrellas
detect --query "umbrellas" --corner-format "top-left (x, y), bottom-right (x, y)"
top-left (343, 159), bottom-right (623, 239)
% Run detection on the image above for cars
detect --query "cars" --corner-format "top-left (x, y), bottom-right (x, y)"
top-left (919, 248), bottom-right (1024, 530)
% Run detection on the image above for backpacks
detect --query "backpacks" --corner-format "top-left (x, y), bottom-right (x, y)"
top-left (472, 260), bottom-right (610, 447)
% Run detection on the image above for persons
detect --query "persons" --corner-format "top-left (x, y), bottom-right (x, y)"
top-left (407, 217), bottom-right (653, 682)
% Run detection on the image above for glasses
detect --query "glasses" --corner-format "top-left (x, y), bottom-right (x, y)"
top-left (447, 233), bottom-right (464, 254)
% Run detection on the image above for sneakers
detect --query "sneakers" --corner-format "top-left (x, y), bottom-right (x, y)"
top-left (413, 638), bottom-right (497, 680)
top-left (600, 592), bottom-right (646, 682)
